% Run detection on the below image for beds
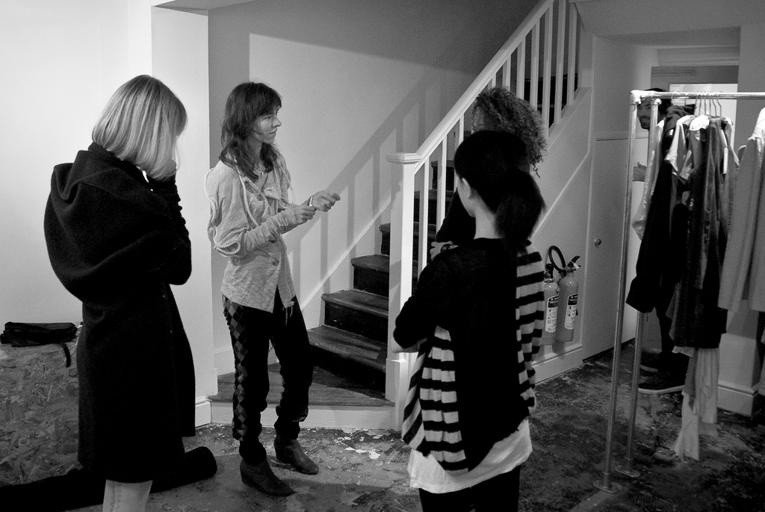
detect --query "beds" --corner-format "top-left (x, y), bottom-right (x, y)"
top-left (2, 323), bottom-right (101, 512)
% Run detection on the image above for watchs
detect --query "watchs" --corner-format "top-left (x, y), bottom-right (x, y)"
top-left (439, 243), bottom-right (452, 252)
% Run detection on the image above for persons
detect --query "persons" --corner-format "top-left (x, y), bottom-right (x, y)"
top-left (628, 84), bottom-right (706, 400)
top-left (42, 74), bottom-right (195, 512)
top-left (205, 81), bottom-right (340, 498)
top-left (393, 129), bottom-right (546, 512)
top-left (431, 87), bottom-right (548, 258)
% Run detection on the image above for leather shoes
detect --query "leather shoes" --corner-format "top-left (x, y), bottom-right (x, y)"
top-left (275, 436), bottom-right (321, 476)
top-left (240, 460), bottom-right (296, 497)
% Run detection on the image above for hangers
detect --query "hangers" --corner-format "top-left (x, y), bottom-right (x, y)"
top-left (664, 93), bottom-right (728, 129)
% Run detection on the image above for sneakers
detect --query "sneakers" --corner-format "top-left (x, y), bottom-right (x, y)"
top-left (638, 352), bottom-right (682, 373)
top-left (636, 368), bottom-right (688, 396)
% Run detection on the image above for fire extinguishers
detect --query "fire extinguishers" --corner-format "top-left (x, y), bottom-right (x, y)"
top-left (550, 246), bottom-right (581, 343)
top-left (545, 264), bottom-right (558, 345)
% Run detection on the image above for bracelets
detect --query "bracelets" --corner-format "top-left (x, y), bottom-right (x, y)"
top-left (308, 197), bottom-right (313, 207)
